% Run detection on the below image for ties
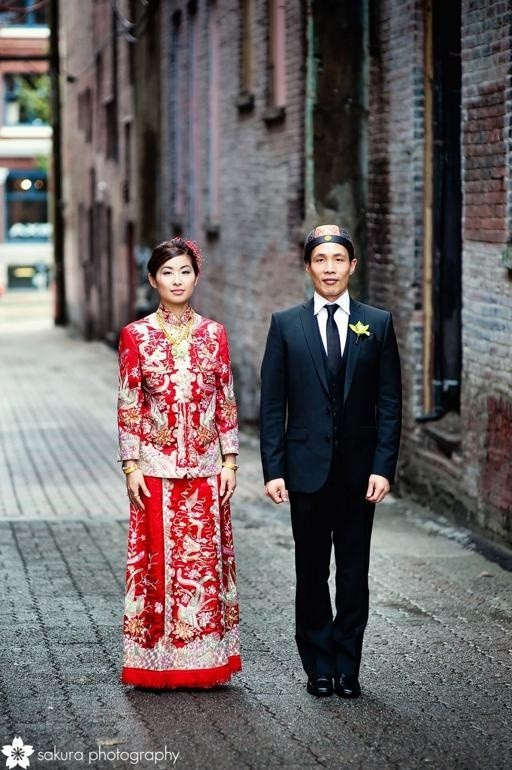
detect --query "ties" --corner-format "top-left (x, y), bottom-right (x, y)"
top-left (322, 303), bottom-right (345, 398)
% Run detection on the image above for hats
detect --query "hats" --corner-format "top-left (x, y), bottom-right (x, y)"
top-left (303, 223), bottom-right (354, 258)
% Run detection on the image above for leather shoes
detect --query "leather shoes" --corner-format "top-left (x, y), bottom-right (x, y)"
top-left (306, 674), bottom-right (334, 698)
top-left (333, 672), bottom-right (361, 699)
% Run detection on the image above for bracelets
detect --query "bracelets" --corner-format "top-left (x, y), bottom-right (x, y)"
top-left (222, 461), bottom-right (238, 471)
top-left (122, 464), bottom-right (138, 475)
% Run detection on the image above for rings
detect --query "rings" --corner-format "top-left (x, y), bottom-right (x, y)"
top-left (134, 494), bottom-right (139, 497)
top-left (227, 489), bottom-right (234, 494)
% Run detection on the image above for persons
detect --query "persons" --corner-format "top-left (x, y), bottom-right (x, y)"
top-left (258, 223), bottom-right (402, 697)
top-left (118, 236), bottom-right (243, 691)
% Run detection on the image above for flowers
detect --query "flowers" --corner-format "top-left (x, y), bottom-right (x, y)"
top-left (349, 319), bottom-right (370, 344)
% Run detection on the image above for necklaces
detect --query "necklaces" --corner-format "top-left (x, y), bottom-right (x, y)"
top-left (153, 310), bottom-right (194, 356)
